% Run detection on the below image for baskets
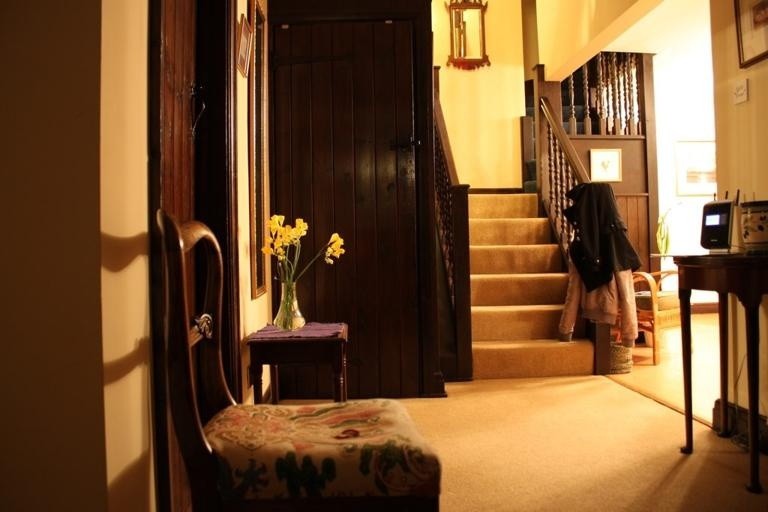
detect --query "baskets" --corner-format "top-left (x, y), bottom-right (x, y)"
top-left (609, 339), bottom-right (634, 374)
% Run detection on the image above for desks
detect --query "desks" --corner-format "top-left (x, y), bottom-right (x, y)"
top-left (673, 252), bottom-right (768, 494)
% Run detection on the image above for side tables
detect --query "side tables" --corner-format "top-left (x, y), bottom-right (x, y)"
top-left (248, 323), bottom-right (348, 406)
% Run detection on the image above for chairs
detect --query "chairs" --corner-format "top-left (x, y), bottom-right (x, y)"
top-left (631, 271), bottom-right (681, 365)
top-left (155, 206), bottom-right (440, 512)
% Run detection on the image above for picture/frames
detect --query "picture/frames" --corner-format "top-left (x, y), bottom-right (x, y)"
top-left (733, 0), bottom-right (768, 68)
top-left (589, 149), bottom-right (623, 183)
top-left (238, 14), bottom-right (253, 77)
top-left (674, 139), bottom-right (717, 197)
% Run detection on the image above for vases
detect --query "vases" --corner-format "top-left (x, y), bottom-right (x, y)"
top-left (274, 282), bottom-right (306, 334)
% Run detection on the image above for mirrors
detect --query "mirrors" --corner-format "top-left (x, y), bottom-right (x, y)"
top-left (249, 0), bottom-right (265, 299)
top-left (448, 0), bottom-right (490, 70)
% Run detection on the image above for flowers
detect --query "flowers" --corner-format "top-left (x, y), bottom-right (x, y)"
top-left (259, 214), bottom-right (346, 331)
